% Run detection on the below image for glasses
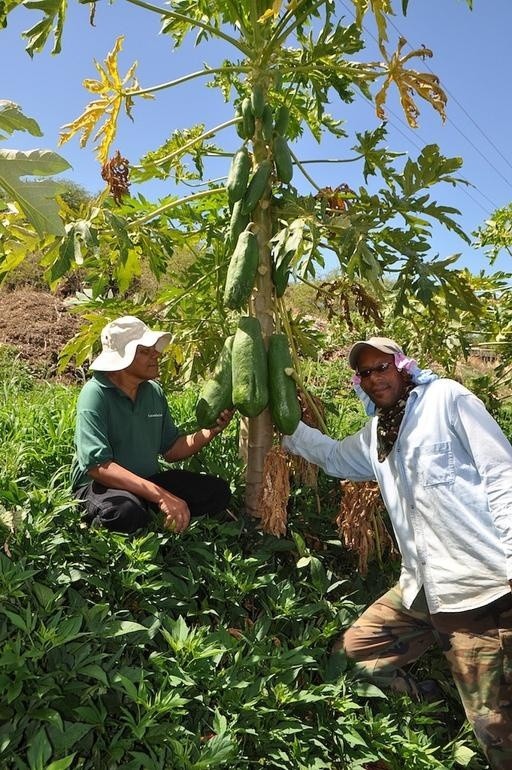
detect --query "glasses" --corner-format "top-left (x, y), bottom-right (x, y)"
top-left (356, 362), bottom-right (394, 379)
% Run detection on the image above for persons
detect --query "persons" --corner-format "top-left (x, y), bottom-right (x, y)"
top-left (277, 336), bottom-right (512, 770)
top-left (68, 314), bottom-right (235, 533)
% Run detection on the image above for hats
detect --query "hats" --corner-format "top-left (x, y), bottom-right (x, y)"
top-left (348, 336), bottom-right (404, 369)
top-left (88, 316), bottom-right (172, 371)
